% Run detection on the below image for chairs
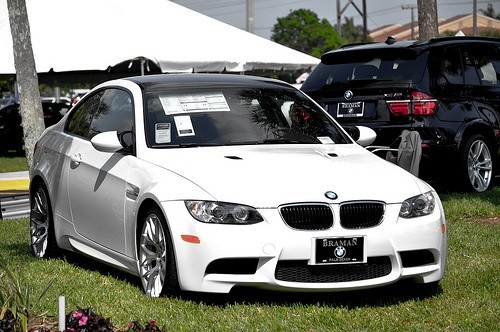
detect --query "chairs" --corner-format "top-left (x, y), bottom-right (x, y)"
top-left (346, 65), bottom-right (379, 79)
top-left (148, 111), bottom-right (219, 146)
top-left (465, 65), bottom-right (484, 85)
top-left (102, 109), bottom-right (157, 133)
top-left (364, 130), bottom-right (422, 177)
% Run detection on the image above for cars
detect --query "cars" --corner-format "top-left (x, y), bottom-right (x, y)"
top-left (0, 103), bottom-right (70, 154)
top-left (28, 72), bottom-right (448, 301)
top-left (0, 88), bottom-right (91, 109)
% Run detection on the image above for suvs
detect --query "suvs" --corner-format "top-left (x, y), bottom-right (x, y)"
top-left (291, 34), bottom-right (500, 194)
